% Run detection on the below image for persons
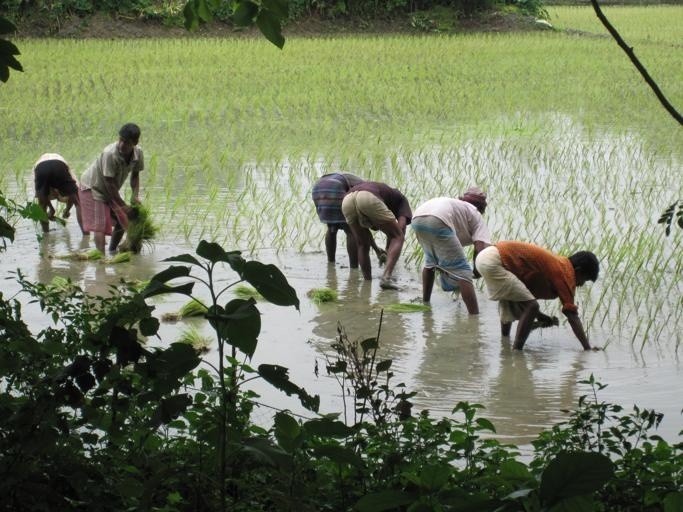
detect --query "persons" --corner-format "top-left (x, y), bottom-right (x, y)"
top-left (76, 123), bottom-right (145, 256)
top-left (410, 188), bottom-right (487, 314)
top-left (310, 172), bottom-right (365, 269)
top-left (31, 153), bottom-right (79, 233)
top-left (340, 182), bottom-right (412, 290)
top-left (475, 240), bottom-right (600, 350)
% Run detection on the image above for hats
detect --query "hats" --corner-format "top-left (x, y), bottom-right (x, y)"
top-left (459, 187), bottom-right (488, 212)
